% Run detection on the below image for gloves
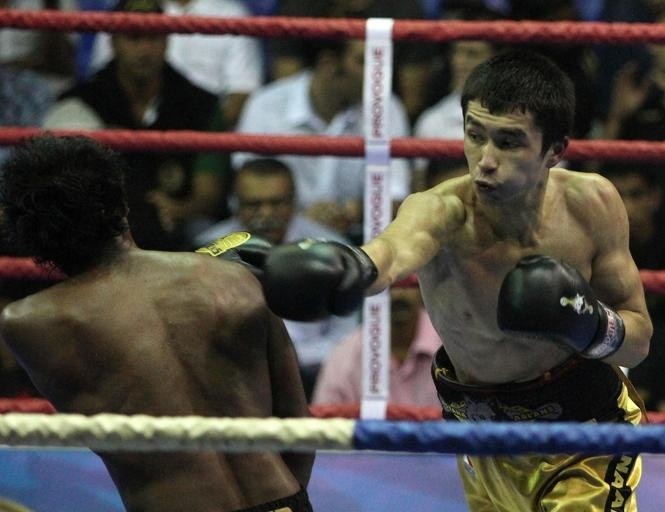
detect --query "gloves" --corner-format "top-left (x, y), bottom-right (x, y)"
top-left (262, 238), bottom-right (378, 322)
top-left (497, 254), bottom-right (625, 360)
top-left (195, 232), bottom-right (272, 281)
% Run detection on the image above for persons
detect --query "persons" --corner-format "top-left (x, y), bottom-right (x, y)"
top-left (261, 45), bottom-right (655, 511)
top-left (0, 133), bottom-right (317, 511)
